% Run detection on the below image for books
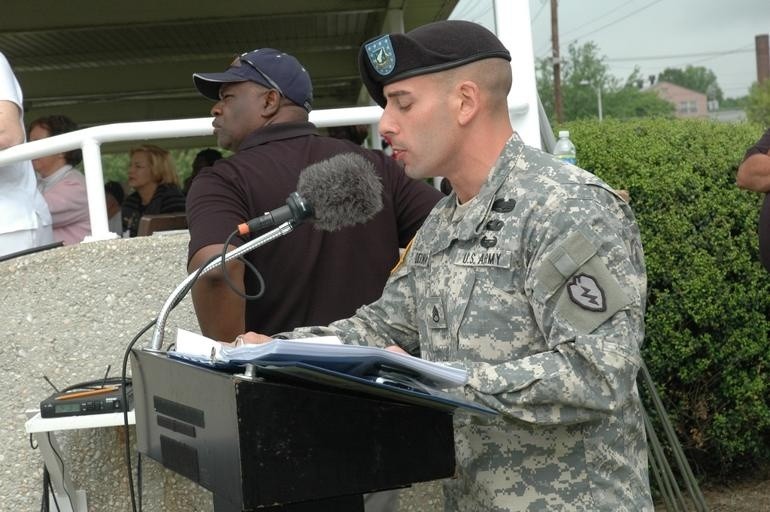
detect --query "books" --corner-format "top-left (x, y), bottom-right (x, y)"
top-left (166, 328), bottom-right (501, 416)
top-left (203, 338), bottom-right (471, 388)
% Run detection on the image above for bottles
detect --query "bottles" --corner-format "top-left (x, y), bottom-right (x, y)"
top-left (551, 130), bottom-right (577, 167)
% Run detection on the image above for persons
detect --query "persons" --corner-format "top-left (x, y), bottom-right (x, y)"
top-left (183, 47), bottom-right (453, 341)
top-left (27, 114), bottom-right (224, 246)
top-left (735, 128), bottom-right (770, 276)
top-left (0, 51), bottom-right (54, 258)
top-left (218, 18), bottom-right (659, 512)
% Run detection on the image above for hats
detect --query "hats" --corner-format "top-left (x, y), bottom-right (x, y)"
top-left (191, 47), bottom-right (315, 115)
top-left (356, 16), bottom-right (512, 111)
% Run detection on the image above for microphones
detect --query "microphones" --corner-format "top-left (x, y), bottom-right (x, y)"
top-left (237, 150), bottom-right (387, 236)
